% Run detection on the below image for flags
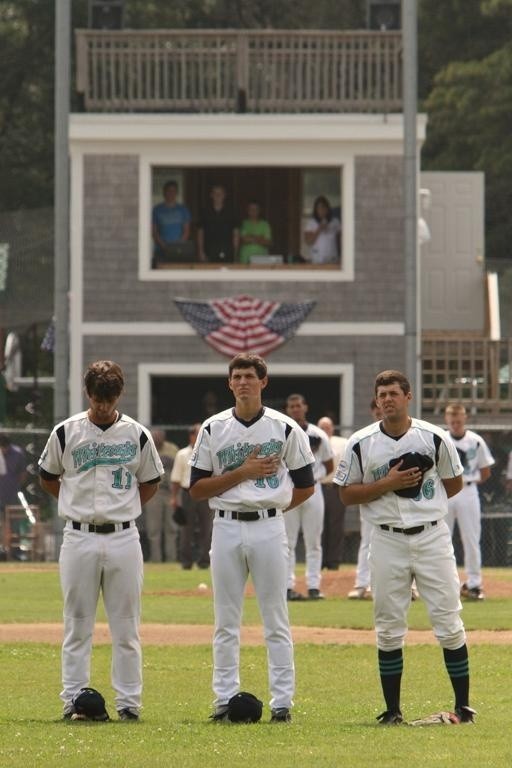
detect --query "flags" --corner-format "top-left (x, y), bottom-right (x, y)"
top-left (169, 294), bottom-right (246, 361)
top-left (246, 295), bottom-right (318, 357)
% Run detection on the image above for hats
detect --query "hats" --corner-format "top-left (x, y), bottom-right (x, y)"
top-left (389, 452), bottom-right (434, 497)
top-left (208, 692), bottom-right (262, 723)
top-left (72, 687), bottom-right (110, 722)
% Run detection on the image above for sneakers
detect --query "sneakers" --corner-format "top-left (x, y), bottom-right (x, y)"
top-left (375, 711), bottom-right (402, 728)
top-left (287, 589), bottom-right (304, 600)
top-left (270, 708), bottom-right (292, 723)
top-left (118, 706), bottom-right (141, 722)
top-left (455, 707), bottom-right (477, 724)
top-left (348, 587), bottom-right (366, 599)
top-left (64, 711), bottom-right (86, 721)
top-left (308, 590), bottom-right (325, 599)
top-left (461, 584), bottom-right (486, 600)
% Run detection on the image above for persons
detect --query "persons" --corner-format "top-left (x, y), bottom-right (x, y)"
top-left (442, 405), bottom-right (512, 601)
top-left (1, 424), bottom-right (215, 568)
top-left (151, 167), bottom-right (343, 271)
top-left (282, 394), bottom-right (421, 601)
top-left (35, 359), bottom-right (165, 722)
top-left (332, 366), bottom-right (471, 723)
top-left (187, 351), bottom-right (316, 724)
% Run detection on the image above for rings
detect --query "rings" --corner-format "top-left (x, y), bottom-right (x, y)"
top-left (408, 470), bottom-right (415, 476)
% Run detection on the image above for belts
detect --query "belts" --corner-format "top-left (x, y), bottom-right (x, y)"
top-left (219, 508), bottom-right (276, 521)
top-left (381, 522), bottom-right (437, 535)
top-left (73, 520), bottom-right (130, 534)
